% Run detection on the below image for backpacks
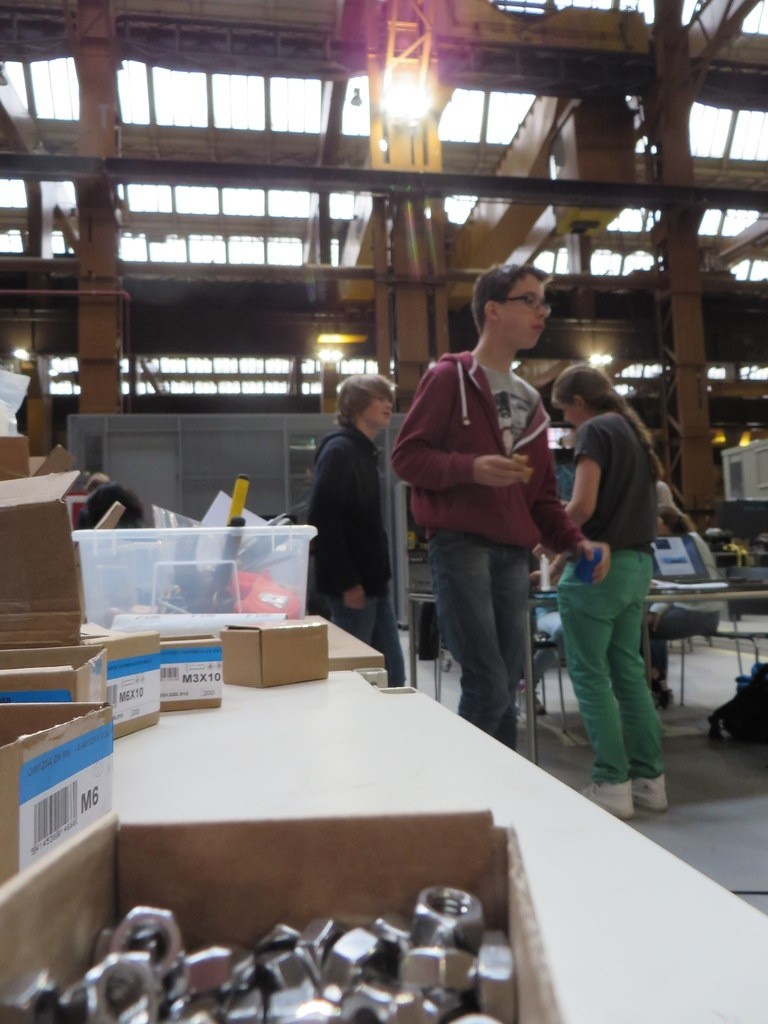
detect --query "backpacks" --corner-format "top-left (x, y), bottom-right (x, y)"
top-left (705, 664), bottom-right (768, 740)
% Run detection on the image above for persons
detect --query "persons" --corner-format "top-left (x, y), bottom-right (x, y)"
top-left (531, 360), bottom-right (670, 818)
top-left (85, 483), bottom-right (188, 630)
top-left (390, 258), bottom-right (608, 749)
top-left (305, 370), bottom-right (409, 691)
top-left (646, 502), bottom-right (725, 710)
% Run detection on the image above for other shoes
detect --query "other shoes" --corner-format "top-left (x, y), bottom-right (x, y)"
top-left (649, 675), bottom-right (675, 711)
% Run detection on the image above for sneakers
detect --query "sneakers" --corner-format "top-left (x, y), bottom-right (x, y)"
top-left (575, 778), bottom-right (637, 821)
top-left (632, 774), bottom-right (668, 812)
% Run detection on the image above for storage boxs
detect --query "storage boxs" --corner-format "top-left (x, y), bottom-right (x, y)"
top-left (2, 434), bottom-right (564, 1023)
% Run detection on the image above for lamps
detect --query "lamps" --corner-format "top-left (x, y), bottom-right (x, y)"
top-left (377, 67), bottom-right (435, 130)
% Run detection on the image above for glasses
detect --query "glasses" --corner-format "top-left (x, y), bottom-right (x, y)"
top-left (505, 293), bottom-right (551, 310)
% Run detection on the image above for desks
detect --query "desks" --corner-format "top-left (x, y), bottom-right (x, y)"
top-left (643, 589), bottom-right (767, 711)
